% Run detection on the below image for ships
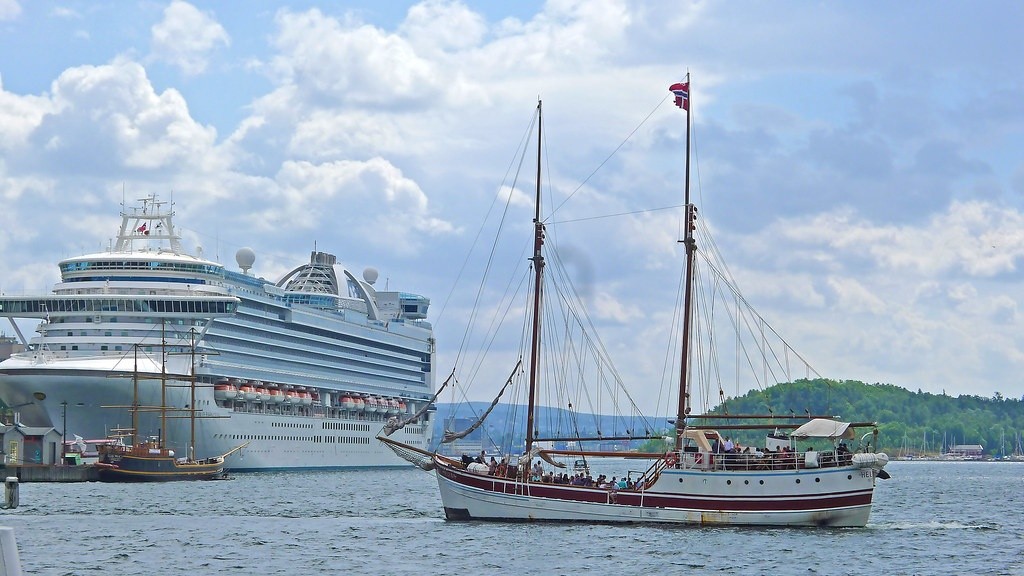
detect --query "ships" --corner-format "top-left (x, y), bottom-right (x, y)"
top-left (1, 181), bottom-right (440, 473)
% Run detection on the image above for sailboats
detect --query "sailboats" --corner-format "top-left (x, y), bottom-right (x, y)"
top-left (92, 317), bottom-right (226, 481)
top-left (376, 97), bottom-right (895, 530)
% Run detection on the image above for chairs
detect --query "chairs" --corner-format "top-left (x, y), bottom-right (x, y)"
top-left (761, 457), bottom-right (771, 470)
top-left (784, 457), bottom-right (800, 470)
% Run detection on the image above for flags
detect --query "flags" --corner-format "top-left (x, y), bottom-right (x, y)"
top-left (668, 81), bottom-right (690, 110)
top-left (136, 223), bottom-right (146, 232)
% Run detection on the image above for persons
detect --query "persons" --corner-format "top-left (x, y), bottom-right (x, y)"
top-left (531, 461), bottom-right (543, 483)
top-left (723, 436), bottom-right (734, 471)
top-left (735, 446), bottom-right (813, 468)
top-left (546, 471), bottom-right (642, 491)
top-left (477, 450), bottom-right (488, 464)
top-left (497, 458), bottom-right (506, 477)
top-left (837, 443), bottom-right (851, 462)
top-left (489, 457), bottom-right (498, 475)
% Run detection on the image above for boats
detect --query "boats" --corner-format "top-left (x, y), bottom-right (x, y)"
top-left (377, 399), bottom-right (388, 413)
top-left (363, 397), bottom-right (377, 412)
top-left (340, 396), bottom-right (354, 409)
top-left (214, 384), bottom-right (237, 400)
top-left (240, 386), bottom-right (258, 401)
top-left (271, 389), bottom-right (285, 404)
top-left (300, 392), bottom-right (312, 406)
top-left (286, 391), bottom-right (300, 405)
top-left (256, 387), bottom-right (270, 402)
top-left (399, 404), bottom-right (408, 414)
top-left (312, 393), bottom-right (321, 404)
top-left (388, 400), bottom-right (400, 415)
top-left (353, 397), bottom-right (364, 411)
